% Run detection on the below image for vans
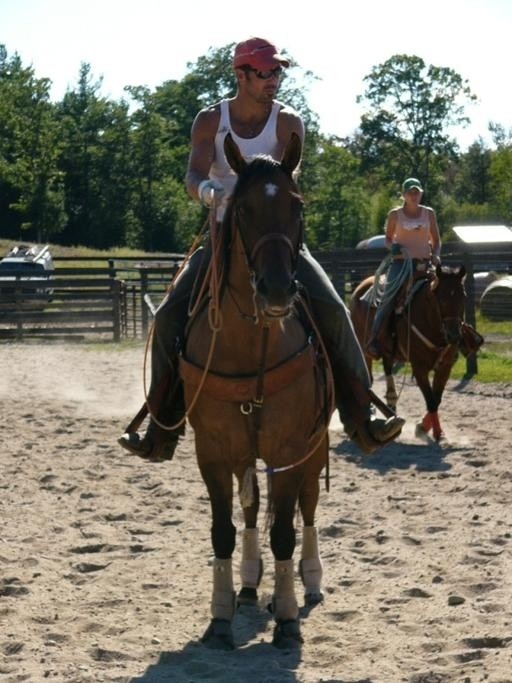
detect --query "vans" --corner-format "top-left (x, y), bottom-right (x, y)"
top-left (0, 243), bottom-right (54, 312)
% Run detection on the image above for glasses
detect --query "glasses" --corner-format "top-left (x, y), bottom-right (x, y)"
top-left (248, 68), bottom-right (282, 80)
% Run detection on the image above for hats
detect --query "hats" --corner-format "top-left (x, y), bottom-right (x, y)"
top-left (233, 36), bottom-right (289, 72)
top-left (402, 177), bottom-right (423, 192)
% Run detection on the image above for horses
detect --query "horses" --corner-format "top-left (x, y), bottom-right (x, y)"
top-left (178, 128), bottom-right (332, 653)
top-left (346, 259), bottom-right (470, 447)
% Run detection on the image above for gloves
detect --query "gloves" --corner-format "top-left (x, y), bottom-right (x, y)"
top-left (431, 255), bottom-right (441, 265)
top-left (391, 243), bottom-right (402, 256)
top-left (198, 179), bottom-right (225, 209)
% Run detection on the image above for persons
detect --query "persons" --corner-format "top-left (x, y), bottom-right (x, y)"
top-left (365, 176), bottom-right (463, 360)
top-left (117, 36), bottom-right (406, 463)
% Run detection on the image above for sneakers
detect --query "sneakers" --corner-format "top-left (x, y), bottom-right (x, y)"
top-left (365, 342), bottom-right (378, 359)
top-left (351, 416), bottom-right (405, 456)
top-left (461, 337), bottom-right (482, 360)
top-left (116, 429), bottom-right (178, 460)
